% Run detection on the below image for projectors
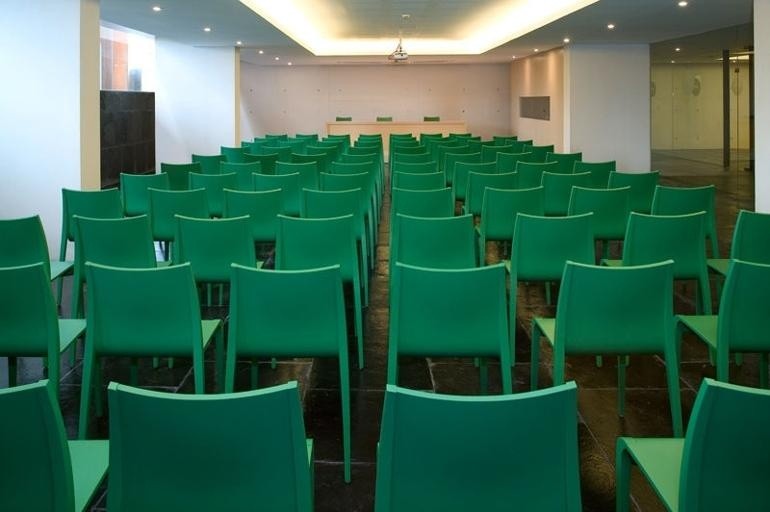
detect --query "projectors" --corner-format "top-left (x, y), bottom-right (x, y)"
top-left (393, 51), bottom-right (408, 59)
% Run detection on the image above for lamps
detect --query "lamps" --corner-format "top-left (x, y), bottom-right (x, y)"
top-left (390, 20), bottom-right (412, 63)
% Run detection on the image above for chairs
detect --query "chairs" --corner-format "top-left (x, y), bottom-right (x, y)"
top-left (389, 262), bottom-right (514, 398)
top-left (527, 261), bottom-right (681, 439)
top-left (0, 215), bottom-right (50, 280)
top-left (614, 373), bottom-right (768, 511)
top-left (55, 113), bottom-right (719, 308)
top-left (221, 263), bottom-right (352, 457)
top-left (0, 372), bottom-right (110, 512)
top-left (102, 379), bottom-right (317, 511)
top-left (372, 377), bottom-right (585, 510)
top-left (681, 260), bottom-right (768, 380)
top-left (0, 263), bottom-right (86, 395)
top-left (708, 209), bottom-right (770, 275)
top-left (79, 261), bottom-right (224, 440)
